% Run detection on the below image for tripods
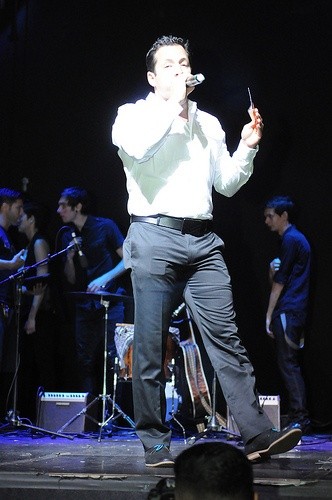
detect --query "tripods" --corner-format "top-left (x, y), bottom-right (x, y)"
top-left (0, 242), bottom-right (136, 442)
top-left (187, 370), bottom-right (243, 445)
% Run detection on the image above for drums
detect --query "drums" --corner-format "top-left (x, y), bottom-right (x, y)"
top-left (162, 363), bottom-right (186, 426)
top-left (113, 323), bottom-right (180, 381)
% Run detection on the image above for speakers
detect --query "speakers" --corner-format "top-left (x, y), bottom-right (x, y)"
top-left (226, 395), bottom-right (280, 439)
top-left (39, 392), bottom-right (97, 433)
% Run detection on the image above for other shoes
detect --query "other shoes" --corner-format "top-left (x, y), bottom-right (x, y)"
top-left (144, 444), bottom-right (176, 467)
top-left (282, 424), bottom-right (311, 435)
top-left (245, 429), bottom-right (302, 463)
top-left (280, 420), bottom-right (288, 429)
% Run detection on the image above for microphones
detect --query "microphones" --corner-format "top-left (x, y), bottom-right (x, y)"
top-left (171, 302), bottom-right (185, 320)
top-left (185, 72), bottom-right (204, 86)
top-left (71, 230), bottom-right (83, 256)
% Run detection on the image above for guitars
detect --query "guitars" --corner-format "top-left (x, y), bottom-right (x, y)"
top-left (178, 303), bottom-right (213, 418)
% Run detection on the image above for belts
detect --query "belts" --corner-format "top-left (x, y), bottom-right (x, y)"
top-left (130, 215), bottom-right (212, 237)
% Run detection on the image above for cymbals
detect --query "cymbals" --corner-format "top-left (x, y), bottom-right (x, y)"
top-left (68, 291), bottom-right (132, 303)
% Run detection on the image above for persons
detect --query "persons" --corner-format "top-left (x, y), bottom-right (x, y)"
top-left (56, 186), bottom-right (130, 433)
top-left (0, 186), bottom-right (51, 435)
top-left (110, 34), bottom-right (303, 466)
top-left (262, 196), bottom-right (314, 428)
top-left (176, 442), bottom-right (258, 500)
